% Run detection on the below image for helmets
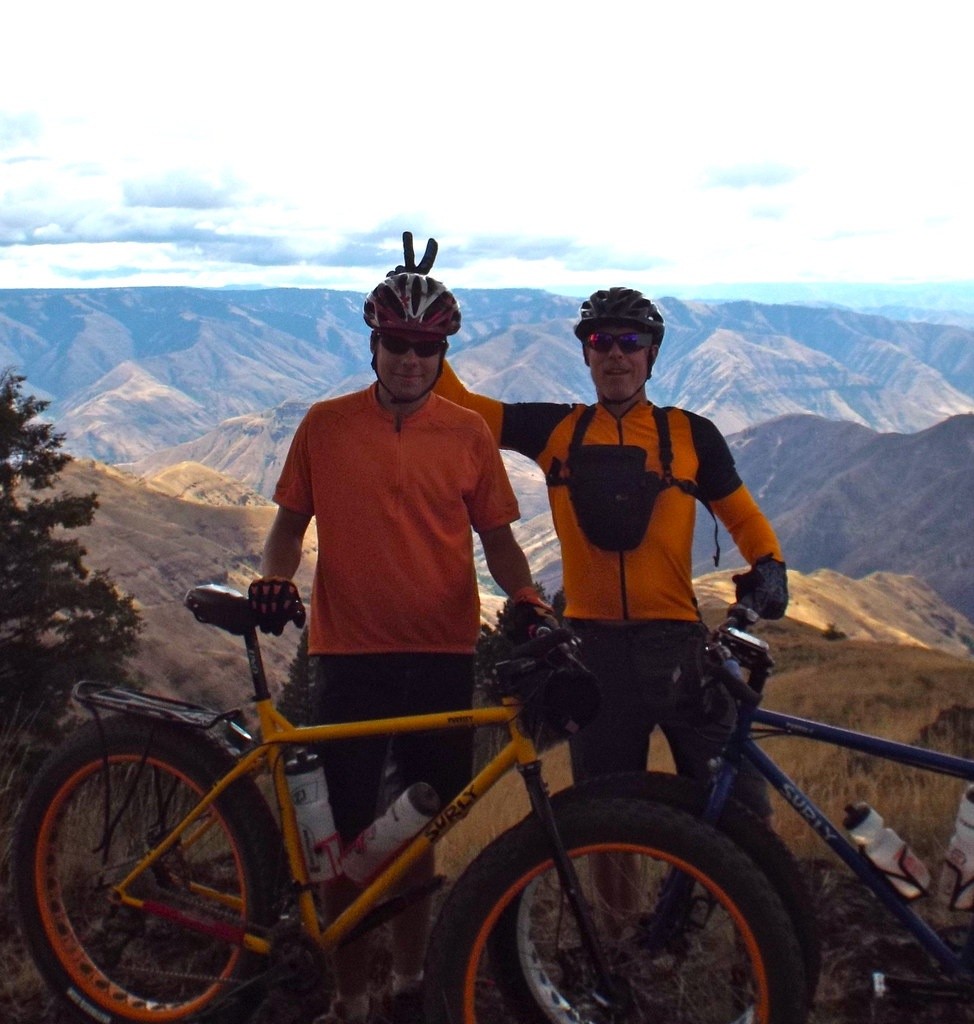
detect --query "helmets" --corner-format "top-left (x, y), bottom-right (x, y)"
top-left (575, 286), bottom-right (666, 346)
top-left (363, 271), bottom-right (461, 335)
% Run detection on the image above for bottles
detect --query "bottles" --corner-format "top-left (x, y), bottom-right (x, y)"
top-left (340, 783), bottom-right (441, 885)
top-left (287, 750), bottom-right (342, 882)
top-left (940, 785), bottom-right (974, 909)
top-left (843, 801), bottom-right (929, 898)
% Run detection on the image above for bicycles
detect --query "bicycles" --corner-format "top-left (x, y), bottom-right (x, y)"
top-left (9, 585), bottom-right (810, 1024)
top-left (484, 593), bottom-right (973, 1024)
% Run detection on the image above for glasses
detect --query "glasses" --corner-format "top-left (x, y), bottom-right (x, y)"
top-left (375, 331), bottom-right (445, 358)
top-left (583, 332), bottom-right (644, 352)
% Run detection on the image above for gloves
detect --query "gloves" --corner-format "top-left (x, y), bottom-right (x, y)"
top-left (511, 587), bottom-right (560, 641)
top-left (248, 576), bottom-right (306, 633)
top-left (386, 232), bottom-right (438, 277)
top-left (732, 552), bottom-right (788, 620)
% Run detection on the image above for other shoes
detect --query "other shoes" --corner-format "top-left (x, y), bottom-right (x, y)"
top-left (589, 943), bottom-right (634, 1021)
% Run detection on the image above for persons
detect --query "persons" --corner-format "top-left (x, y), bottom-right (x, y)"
top-left (250, 268), bottom-right (563, 1023)
top-left (387, 232), bottom-right (788, 1009)
